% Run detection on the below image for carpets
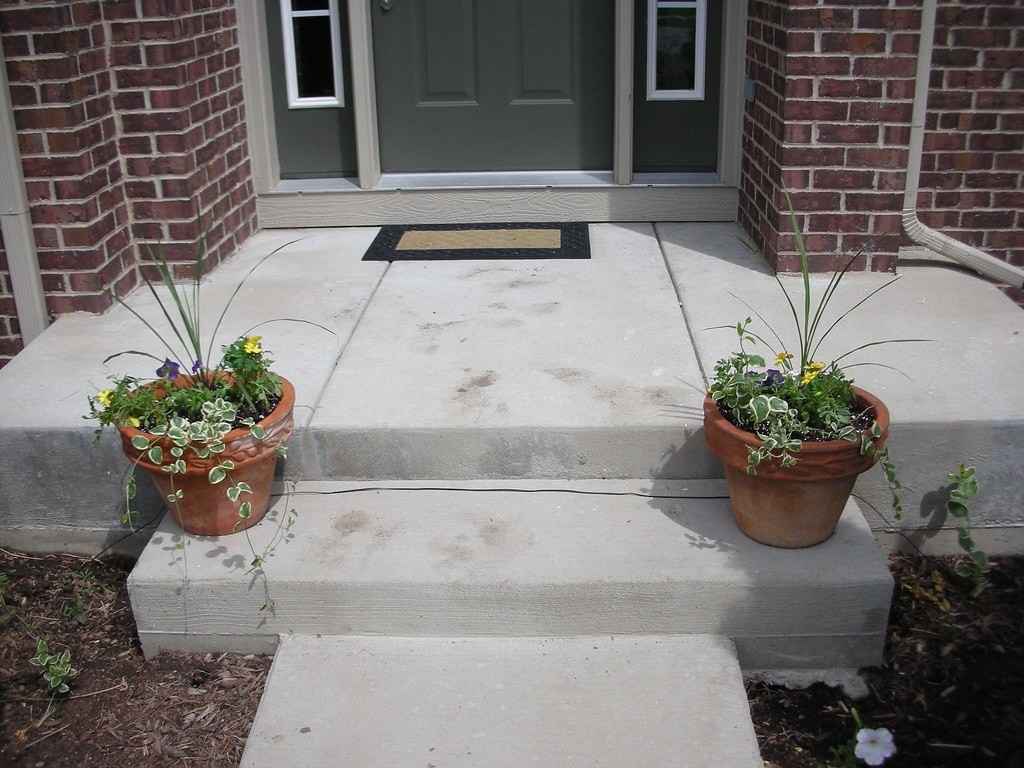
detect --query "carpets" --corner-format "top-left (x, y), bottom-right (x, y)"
top-left (362, 223), bottom-right (591, 261)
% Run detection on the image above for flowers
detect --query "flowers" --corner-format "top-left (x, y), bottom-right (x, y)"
top-left (55, 197), bottom-right (336, 611)
top-left (850, 722), bottom-right (898, 764)
top-left (695, 178), bottom-right (936, 522)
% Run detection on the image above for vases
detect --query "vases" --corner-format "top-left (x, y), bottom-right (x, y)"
top-left (700, 384), bottom-right (890, 550)
top-left (112, 371), bottom-right (296, 536)
